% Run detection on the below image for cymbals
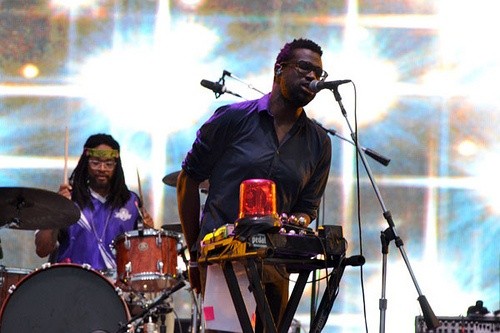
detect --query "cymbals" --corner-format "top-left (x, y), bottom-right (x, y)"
top-left (160, 222), bottom-right (185, 233)
top-left (0, 184), bottom-right (81, 232)
top-left (161, 169), bottom-right (211, 192)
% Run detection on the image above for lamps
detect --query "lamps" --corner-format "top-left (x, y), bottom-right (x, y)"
top-left (235, 179), bottom-right (282, 230)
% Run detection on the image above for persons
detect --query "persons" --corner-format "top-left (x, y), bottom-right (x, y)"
top-left (35, 133), bottom-right (154, 272)
top-left (176, 37), bottom-right (332, 333)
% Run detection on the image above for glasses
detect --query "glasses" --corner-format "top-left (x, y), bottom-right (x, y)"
top-left (88, 160), bottom-right (117, 171)
top-left (280, 61), bottom-right (328, 81)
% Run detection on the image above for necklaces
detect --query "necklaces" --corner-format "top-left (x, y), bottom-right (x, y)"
top-left (88, 195), bottom-right (112, 243)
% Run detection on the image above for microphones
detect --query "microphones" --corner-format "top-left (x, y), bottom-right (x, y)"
top-left (309, 79), bottom-right (352, 93)
top-left (201, 79), bottom-right (225, 94)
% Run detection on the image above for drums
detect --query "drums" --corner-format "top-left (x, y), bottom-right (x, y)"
top-left (0, 262), bottom-right (135, 333)
top-left (0, 268), bottom-right (33, 310)
top-left (112, 228), bottom-right (181, 294)
